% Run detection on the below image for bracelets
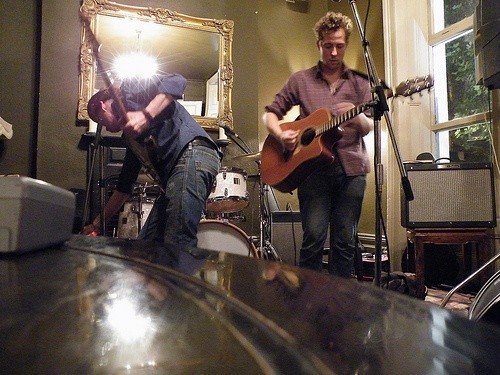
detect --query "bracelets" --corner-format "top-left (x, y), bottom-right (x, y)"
top-left (142, 109), bottom-right (152, 124)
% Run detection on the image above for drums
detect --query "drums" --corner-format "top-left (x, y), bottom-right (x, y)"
top-left (114, 195), bottom-right (157, 242)
top-left (195, 217), bottom-right (260, 258)
top-left (204, 166), bottom-right (251, 215)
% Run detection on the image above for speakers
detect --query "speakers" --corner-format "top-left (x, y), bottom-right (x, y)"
top-left (467, 269), bottom-right (500, 328)
top-left (400, 161), bottom-right (497, 230)
top-left (270, 211), bottom-right (332, 269)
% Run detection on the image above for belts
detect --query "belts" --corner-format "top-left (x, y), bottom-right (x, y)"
top-left (191, 139), bottom-right (208, 146)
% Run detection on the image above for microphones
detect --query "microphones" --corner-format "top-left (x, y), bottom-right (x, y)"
top-left (218, 121), bottom-right (240, 139)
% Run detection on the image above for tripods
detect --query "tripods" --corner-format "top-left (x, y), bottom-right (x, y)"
top-left (229, 138), bottom-right (281, 264)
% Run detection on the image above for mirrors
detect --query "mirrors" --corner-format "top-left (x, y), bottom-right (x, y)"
top-left (77, 0), bottom-right (235, 130)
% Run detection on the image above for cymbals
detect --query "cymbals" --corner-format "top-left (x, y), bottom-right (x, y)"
top-left (230, 150), bottom-right (262, 163)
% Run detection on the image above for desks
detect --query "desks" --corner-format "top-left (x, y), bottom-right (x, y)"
top-left (415, 228), bottom-right (492, 287)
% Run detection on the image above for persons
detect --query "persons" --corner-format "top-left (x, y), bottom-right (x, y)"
top-left (262, 11), bottom-right (374, 279)
top-left (81, 73), bottom-right (223, 248)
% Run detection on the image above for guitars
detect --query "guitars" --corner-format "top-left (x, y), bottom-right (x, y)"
top-left (77, 9), bottom-right (168, 187)
top-left (259, 72), bottom-right (435, 194)
